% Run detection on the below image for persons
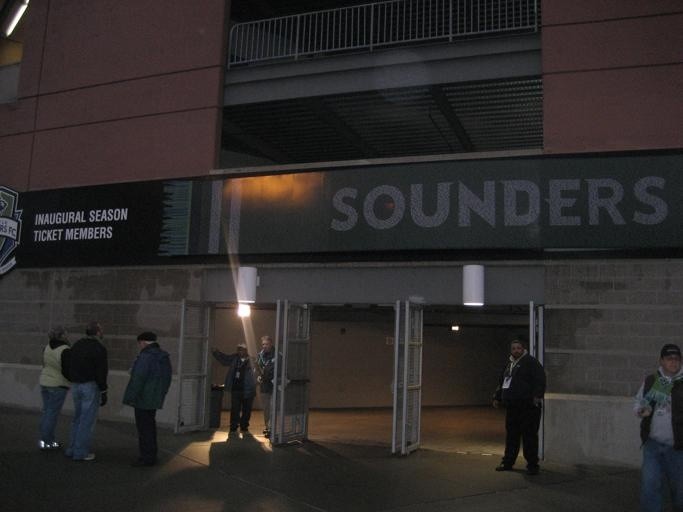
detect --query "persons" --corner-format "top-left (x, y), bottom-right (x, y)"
top-left (633, 343), bottom-right (683, 511)
top-left (65, 321), bottom-right (108, 460)
top-left (38, 324), bottom-right (97, 449)
top-left (492, 340), bottom-right (546, 475)
top-left (123, 332), bottom-right (171, 466)
top-left (257, 335), bottom-right (283, 437)
top-left (209, 343), bottom-right (257, 437)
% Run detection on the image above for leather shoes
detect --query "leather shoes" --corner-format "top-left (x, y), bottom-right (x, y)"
top-left (131, 455), bottom-right (156, 467)
top-left (230, 424), bottom-right (247, 432)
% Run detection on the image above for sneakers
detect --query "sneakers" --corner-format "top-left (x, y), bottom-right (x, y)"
top-left (494, 462), bottom-right (513, 471)
top-left (38, 440), bottom-right (64, 450)
top-left (526, 466), bottom-right (539, 474)
top-left (263, 428), bottom-right (270, 438)
top-left (82, 452), bottom-right (95, 460)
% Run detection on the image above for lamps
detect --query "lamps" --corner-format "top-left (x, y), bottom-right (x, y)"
top-left (462, 265), bottom-right (486, 306)
top-left (237, 266), bottom-right (260, 305)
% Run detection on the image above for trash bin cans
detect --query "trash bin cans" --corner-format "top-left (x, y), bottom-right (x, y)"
top-left (210, 384), bottom-right (224, 428)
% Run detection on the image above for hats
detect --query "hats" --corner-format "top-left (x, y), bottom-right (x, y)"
top-left (235, 343), bottom-right (248, 349)
top-left (136, 332), bottom-right (157, 341)
top-left (660, 343), bottom-right (681, 358)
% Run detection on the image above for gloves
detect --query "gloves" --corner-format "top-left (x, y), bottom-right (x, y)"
top-left (99, 391), bottom-right (107, 406)
